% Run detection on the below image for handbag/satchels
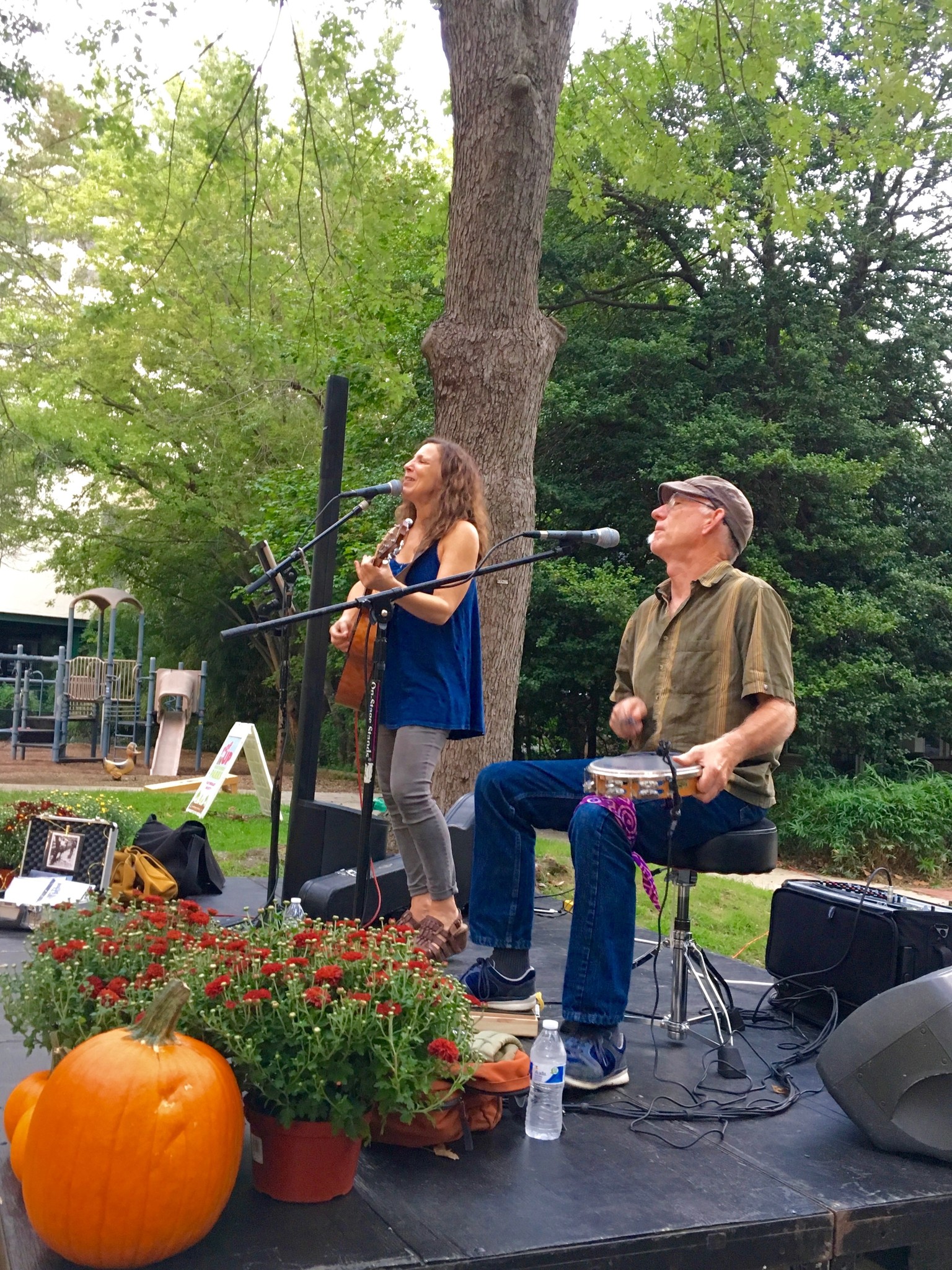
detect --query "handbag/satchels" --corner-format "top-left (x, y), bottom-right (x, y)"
top-left (108, 845), bottom-right (178, 901)
top-left (132, 813), bottom-right (226, 896)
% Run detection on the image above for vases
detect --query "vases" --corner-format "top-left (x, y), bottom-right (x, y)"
top-left (0, 870), bottom-right (19, 891)
top-left (245, 1105), bottom-right (371, 1205)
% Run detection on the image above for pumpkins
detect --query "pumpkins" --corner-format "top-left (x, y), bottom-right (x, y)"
top-left (21, 978), bottom-right (244, 1270)
top-left (4, 1047), bottom-right (71, 1141)
top-left (10, 1105), bottom-right (35, 1181)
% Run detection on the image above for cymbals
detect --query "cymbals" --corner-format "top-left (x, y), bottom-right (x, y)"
top-left (583, 780), bottom-right (659, 800)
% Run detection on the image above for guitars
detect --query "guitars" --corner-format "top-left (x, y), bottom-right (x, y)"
top-left (334, 517), bottom-right (413, 711)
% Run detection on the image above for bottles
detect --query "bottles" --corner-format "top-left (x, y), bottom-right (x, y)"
top-left (524, 1020), bottom-right (568, 1141)
top-left (284, 897), bottom-right (305, 928)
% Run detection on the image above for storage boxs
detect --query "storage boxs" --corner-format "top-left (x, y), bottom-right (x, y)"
top-left (0, 812), bottom-right (119, 930)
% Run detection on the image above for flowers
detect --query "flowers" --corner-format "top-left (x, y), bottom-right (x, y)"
top-left (1, 884), bottom-right (492, 1149)
top-left (0, 790), bottom-right (141, 869)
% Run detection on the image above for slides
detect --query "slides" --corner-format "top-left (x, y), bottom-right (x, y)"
top-left (149, 711), bottom-right (187, 776)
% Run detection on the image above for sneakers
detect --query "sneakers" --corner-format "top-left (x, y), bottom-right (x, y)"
top-left (529, 1020), bottom-right (630, 1090)
top-left (459, 957), bottom-right (536, 1012)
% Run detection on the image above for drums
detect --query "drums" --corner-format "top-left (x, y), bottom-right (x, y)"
top-left (582, 755), bottom-right (703, 800)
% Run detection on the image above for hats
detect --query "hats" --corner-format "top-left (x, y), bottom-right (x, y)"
top-left (657, 476), bottom-right (754, 554)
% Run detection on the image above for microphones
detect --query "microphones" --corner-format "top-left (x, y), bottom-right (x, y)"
top-left (522, 527), bottom-right (620, 549)
top-left (339, 479), bottom-right (404, 499)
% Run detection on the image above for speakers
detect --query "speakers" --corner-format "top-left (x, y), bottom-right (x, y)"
top-left (282, 798), bottom-right (390, 908)
top-left (810, 963), bottom-right (952, 1163)
top-left (765, 878), bottom-right (952, 1031)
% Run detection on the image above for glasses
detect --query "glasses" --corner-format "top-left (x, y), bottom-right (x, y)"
top-left (667, 492), bottom-right (726, 525)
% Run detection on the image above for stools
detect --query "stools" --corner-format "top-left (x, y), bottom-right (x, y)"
top-left (631, 821), bottom-right (780, 1080)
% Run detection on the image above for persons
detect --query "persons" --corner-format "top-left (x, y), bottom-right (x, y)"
top-left (328, 436), bottom-right (486, 965)
top-left (454, 474), bottom-right (797, 1096)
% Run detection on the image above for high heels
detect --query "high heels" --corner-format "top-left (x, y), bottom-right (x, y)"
top-left (395, 907), bottom-right (468, 961)
